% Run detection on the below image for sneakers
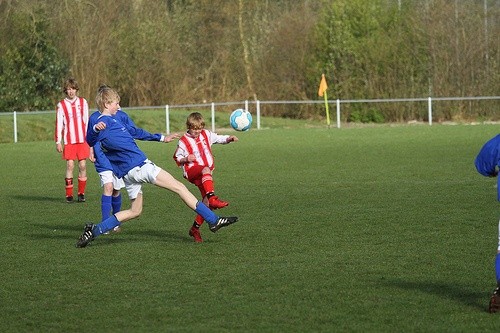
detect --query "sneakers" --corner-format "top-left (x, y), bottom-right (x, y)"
top-left (75, 222), bottom-right (96, 249)
top-left (207, 195), bottom-right (229, 211)
top-left (209, 216), bottom-right (240, 233)
top-left (189, 226), bottom-right (203, 243)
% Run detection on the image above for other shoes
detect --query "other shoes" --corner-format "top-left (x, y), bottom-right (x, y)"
top-left (487, 283), bottom-right (500, 313)
top-left (65, 195), bottom-right (73, 203)
top-left (101, 225), bottom-right (120, 235)
top-left (78, 193), bottom-right (85, 202)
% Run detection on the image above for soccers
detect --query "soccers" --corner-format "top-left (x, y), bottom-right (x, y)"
top-left (229, 108), bottom-right (253, 131)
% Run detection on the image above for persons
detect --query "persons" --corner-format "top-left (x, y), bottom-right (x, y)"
top-left (173, 112), bottom-right (239, 243)
top-left (77, 85), bottom-right (239, 254)
top-left (55, 78), bottom-right (89, 204)
top-left (475, 133), bottom-right (500, 313)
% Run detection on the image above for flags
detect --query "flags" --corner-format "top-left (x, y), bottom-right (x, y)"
top-left (319, 76), bottom-right (328, 96)
